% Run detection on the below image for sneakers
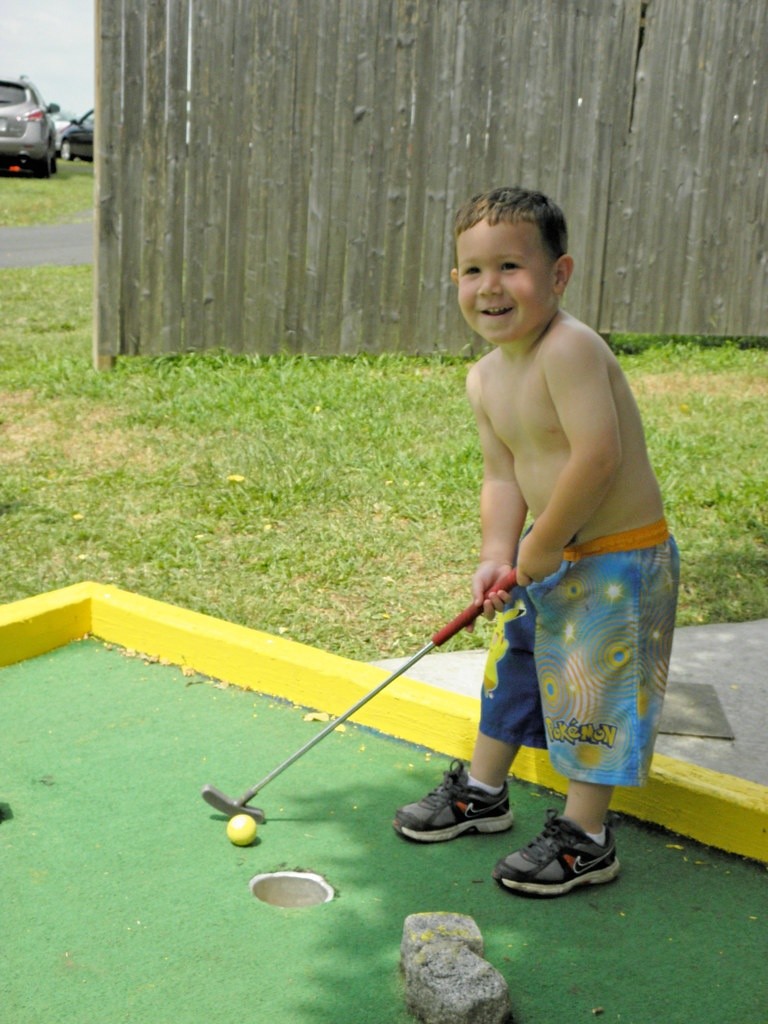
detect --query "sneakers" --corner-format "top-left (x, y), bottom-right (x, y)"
top-left (392, 759), bottom-right (513, 842)
top-left (492, 809), bottom-right (620, 896)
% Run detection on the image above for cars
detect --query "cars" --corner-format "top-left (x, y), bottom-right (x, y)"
top-left (0, 73), bottom-right (60, 177)
top-left (57, 106), bottom-right (93, 162)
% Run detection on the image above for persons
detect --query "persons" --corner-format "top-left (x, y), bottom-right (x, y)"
top-left (388, 188), bottom-right (682, 897)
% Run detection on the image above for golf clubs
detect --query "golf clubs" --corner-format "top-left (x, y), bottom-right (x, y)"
top-left (201, 568), bottom-right (524, 826)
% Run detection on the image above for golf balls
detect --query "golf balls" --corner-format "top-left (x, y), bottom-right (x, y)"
top-left (224, 814), bottom-right (258, 848)
